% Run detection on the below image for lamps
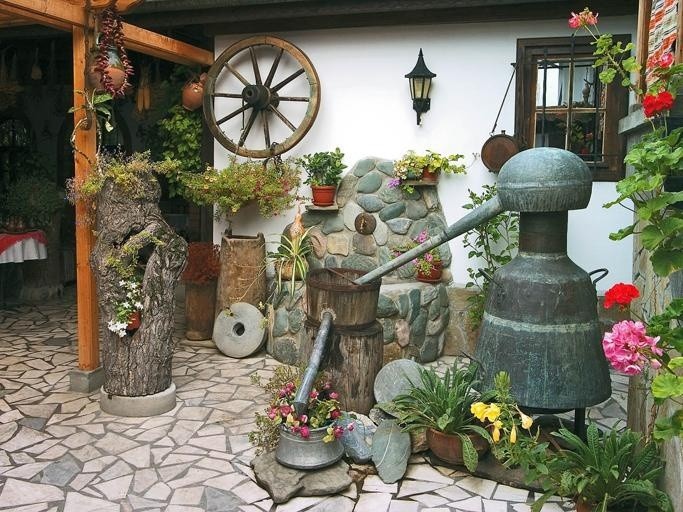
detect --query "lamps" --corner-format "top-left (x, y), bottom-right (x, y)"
top-left (404, 49), bottom-right (436, 126)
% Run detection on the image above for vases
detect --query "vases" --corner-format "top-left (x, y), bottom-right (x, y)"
top-left (574, 492), bottom-right (665, 511)
top-left (421, 166), bottom-right (438, 181)
top-left (122, 309), bottom-right (141, 330)
top-left (274, 420), bottom-right (346, 469)
top-left (415, 259), bottom-right (442, 282)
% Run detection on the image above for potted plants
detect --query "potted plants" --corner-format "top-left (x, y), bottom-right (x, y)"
top-left (234, 225), bottom-right (321, 304)
top-left (373, 354), bottom-right (507, 473)
top-left (296, 145), bottom-right (347, 206)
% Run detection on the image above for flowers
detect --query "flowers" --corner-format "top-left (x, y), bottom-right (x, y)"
top-left (388, 228), bottom-right (442, 278)
top-left (469, 371), bottom-right (665, 512)
top-left (249, 379), bottom-right (351, 449)
top-left (102, 242), bottom-right (144, 340)
top-left (387, 146), bottom-right (479, 195)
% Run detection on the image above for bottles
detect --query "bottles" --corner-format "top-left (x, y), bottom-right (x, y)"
top-left (180, 73), bottom-right (207, 112)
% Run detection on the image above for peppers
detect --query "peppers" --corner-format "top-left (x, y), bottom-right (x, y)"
top-left (94, 3), bottom-right (134, 98)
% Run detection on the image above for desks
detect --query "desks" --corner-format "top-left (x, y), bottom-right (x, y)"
top-left (0, 227), bottom-right (50, 265)
